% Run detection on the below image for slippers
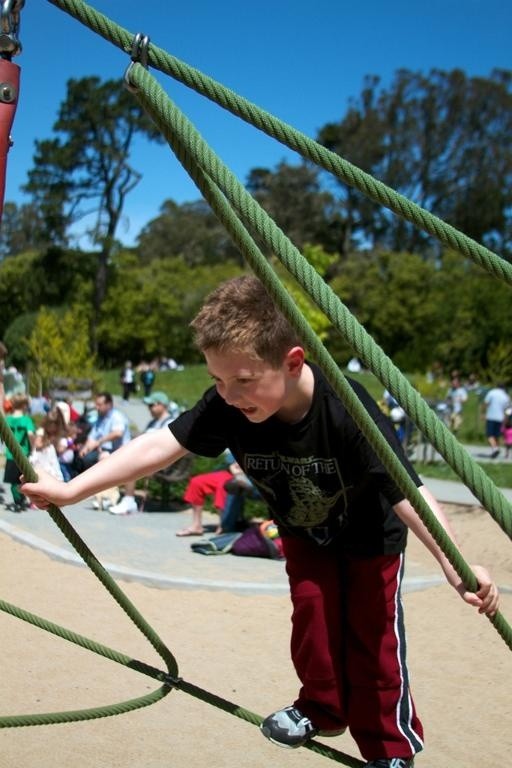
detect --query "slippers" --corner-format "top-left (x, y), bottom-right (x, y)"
top-left (176, 527), bottom-right (203, 536)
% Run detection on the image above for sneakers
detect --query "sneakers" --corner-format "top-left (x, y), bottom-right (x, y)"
top-left (260, 704), bottom-right (347, 749)
top-left (109, 497), bottom-right (137, 514)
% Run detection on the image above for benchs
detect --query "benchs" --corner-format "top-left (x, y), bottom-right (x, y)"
top-left (155, 453), bottom-right (195, 513)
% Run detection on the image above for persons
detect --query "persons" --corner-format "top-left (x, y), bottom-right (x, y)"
top-left (348, 356), bottom-right (511, 466)
top-left (0, 342), bottom-right (280, 537)
top-left (18, 269), bottom-right (498, 768)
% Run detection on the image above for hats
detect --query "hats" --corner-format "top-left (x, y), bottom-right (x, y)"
top-left (144, 391), bottom-right (168, 405)
top-left (55, 401), bottom-right (70, 424)
